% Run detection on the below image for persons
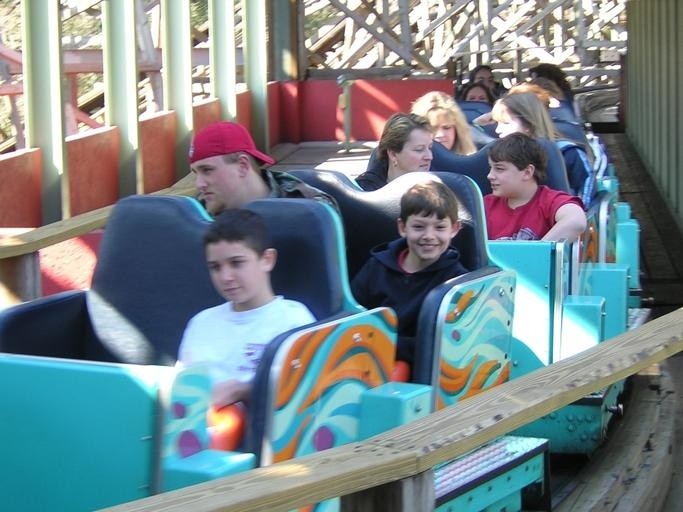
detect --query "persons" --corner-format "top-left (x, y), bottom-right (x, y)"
top-left (410, 91), bottom-right (476, 155)
top-left (482, 132), bottom-right (587, 243)
top-left (354, 112), bottom-right (433, 192)
top-left (350, 180), bottom-right (469, 374)
top-left (189, 121), bottom-right (345, 228)
top-left (176, 208), bottom-right (316, 412)
top-left (492, 92), bottom-right (597, 209)
top-left (469, 65), bottom-right (496, 91)
top-left (461, 82), bottom-right (490, 103)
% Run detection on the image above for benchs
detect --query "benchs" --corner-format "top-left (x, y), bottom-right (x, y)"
top-left (1, 195), bottom-right (433, 512)
top-left (362, 135), bottom-right (638, 456)
top-left (277, 170), bottom-right (548, 505)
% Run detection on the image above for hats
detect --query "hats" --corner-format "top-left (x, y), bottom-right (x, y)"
top-left (187, 120), bottom-right (275, 166)
